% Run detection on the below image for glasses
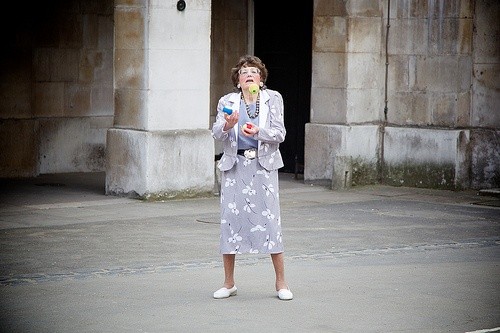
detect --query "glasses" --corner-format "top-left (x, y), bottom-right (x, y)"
top-left (238, 67), bottom-right (261, 75)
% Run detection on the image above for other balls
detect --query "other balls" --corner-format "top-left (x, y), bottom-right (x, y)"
top-left (223, 107), bottom-right (232, 115)
top-left (243, 124), bottom-right (252, 134)
top-left (249, 84), bottom-right (259, 95)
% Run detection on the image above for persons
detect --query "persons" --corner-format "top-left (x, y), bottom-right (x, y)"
top-left (210, 54), bottom-right (294, 302)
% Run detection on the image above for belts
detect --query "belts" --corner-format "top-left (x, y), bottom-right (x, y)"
top-left (237, 149), bottom-right (258, 160)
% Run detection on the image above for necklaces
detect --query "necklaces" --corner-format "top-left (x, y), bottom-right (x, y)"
top-left (238, 91), bottom-right (261, 118)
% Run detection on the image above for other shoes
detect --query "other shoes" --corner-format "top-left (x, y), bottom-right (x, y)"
top-left (213, 285), bottom-right (237, 299)
top-left (275, 286), bottom-right (293, 300)
